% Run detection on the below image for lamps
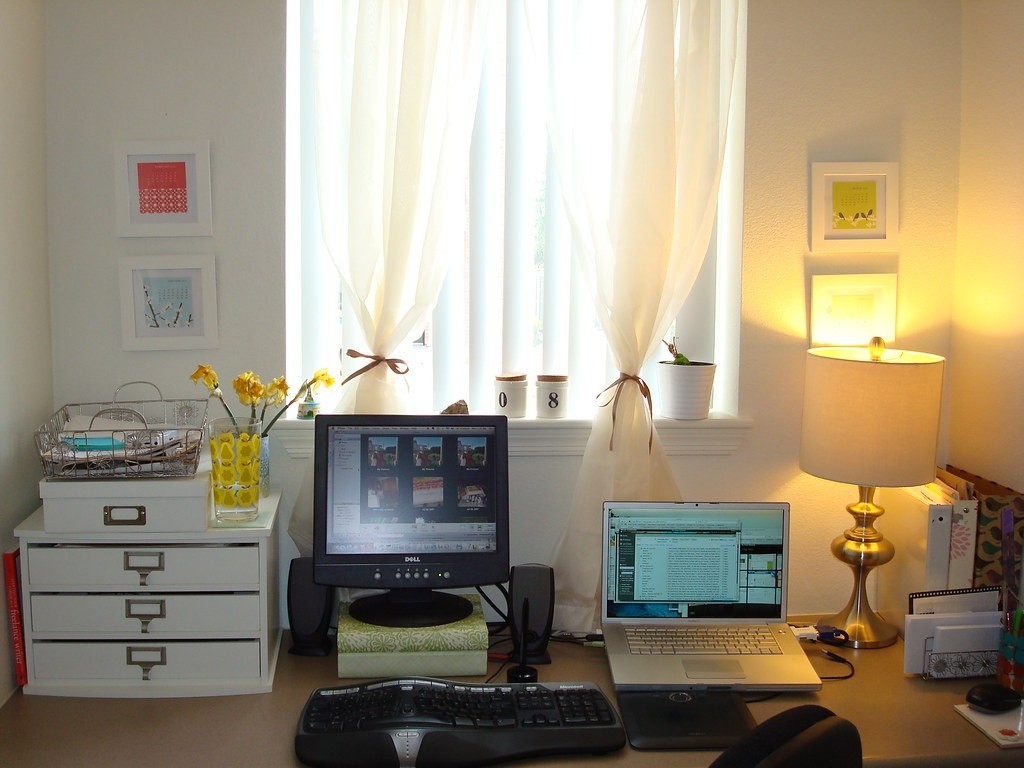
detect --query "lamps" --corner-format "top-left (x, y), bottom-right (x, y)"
top-left (798, 336), bottom-right (944, 650)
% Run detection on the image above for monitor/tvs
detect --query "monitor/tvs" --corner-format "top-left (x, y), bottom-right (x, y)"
top-left (313, 414), bottom-right (510, 628)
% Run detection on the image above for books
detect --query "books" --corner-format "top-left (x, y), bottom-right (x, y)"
top-left (954, 700), bottom-right (1024, 748)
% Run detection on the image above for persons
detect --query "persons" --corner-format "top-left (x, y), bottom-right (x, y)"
top-left (371, 446), bottom-right (386, 466)
top-left (460, 449), bottom-right (473, 470)
top-left (415, 445), bottom-right (429, 471)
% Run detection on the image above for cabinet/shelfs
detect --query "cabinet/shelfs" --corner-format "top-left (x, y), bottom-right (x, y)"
top-left (12, 496), bottom-right (282, 699)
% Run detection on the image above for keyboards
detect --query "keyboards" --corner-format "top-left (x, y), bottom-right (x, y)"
top-left (295, 675), bottom-right (626, 768)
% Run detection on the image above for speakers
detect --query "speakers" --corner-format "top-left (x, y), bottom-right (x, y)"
top-left (287, 557), bottom-right (333, 656)
top-left (508, 562), bottom-right (555, 664)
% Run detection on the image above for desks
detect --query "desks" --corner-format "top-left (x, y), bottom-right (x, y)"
top-left (0, 630), bottom-right (1024, 768)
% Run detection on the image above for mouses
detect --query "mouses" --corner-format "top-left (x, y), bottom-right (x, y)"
top-left (966, 683), bottom-right (1022, 713)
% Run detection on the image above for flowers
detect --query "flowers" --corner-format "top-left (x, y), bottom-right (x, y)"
top-left (189, 362), bottom-right (335, 437)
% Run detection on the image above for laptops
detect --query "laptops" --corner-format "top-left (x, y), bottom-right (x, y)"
top-left (600, 501), bottom-right (822, 692)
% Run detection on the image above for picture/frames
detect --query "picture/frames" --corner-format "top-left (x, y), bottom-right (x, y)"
top-left (117, 254), bottom-right (219, 350)
top-left (811, 161), bottom-right (900, 251)
top-left (114, 139), bottom-right (212, 238)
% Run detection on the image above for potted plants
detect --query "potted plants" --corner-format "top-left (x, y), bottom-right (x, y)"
top-left (655, 334), bottom-right (717, 420)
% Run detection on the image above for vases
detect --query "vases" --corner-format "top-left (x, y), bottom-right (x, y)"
top-left (232, 433), bottom-right (271, 497)
